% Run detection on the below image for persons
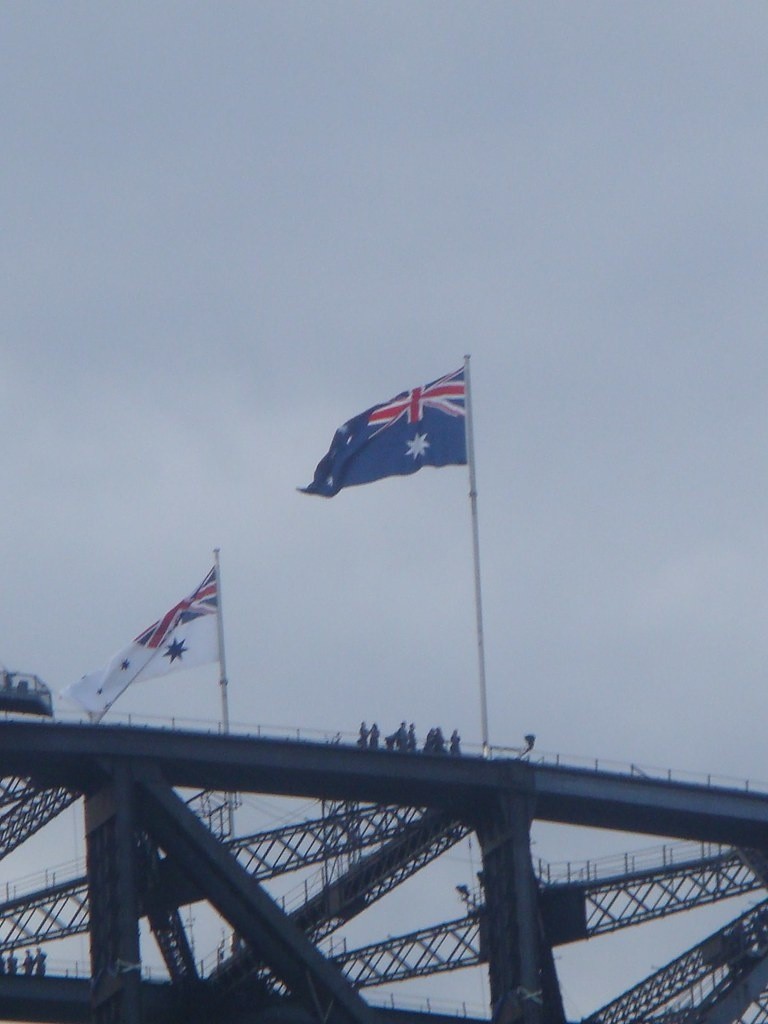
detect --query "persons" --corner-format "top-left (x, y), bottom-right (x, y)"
top-left (0, 951), bottom-right (7, 975)
top-left (450, 730), bottom-right (462, 754)
top-left (482, 741), bottom-right (490, 759)
top-left (7, 949), bottom-right (17, 975)
top-left (396, 721), bottom-right (408, 751)
top-left (22, 949), bottom-right (34, 976)
top-left (423, 726), bottom-right (445, 752)
top-left (367, 724), bottom-right (380, 749)
top-left (33, 948), bottom-right (47, 975)
top-left (408, 723), bottom-right (417, 750)
top-left (358, 722), bottom-right (370, 749)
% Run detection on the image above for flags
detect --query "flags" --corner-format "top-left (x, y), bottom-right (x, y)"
top-left (59, 565), bottom-right (220, 725)
top-left (293, 364), bottom-right (469, 499)
